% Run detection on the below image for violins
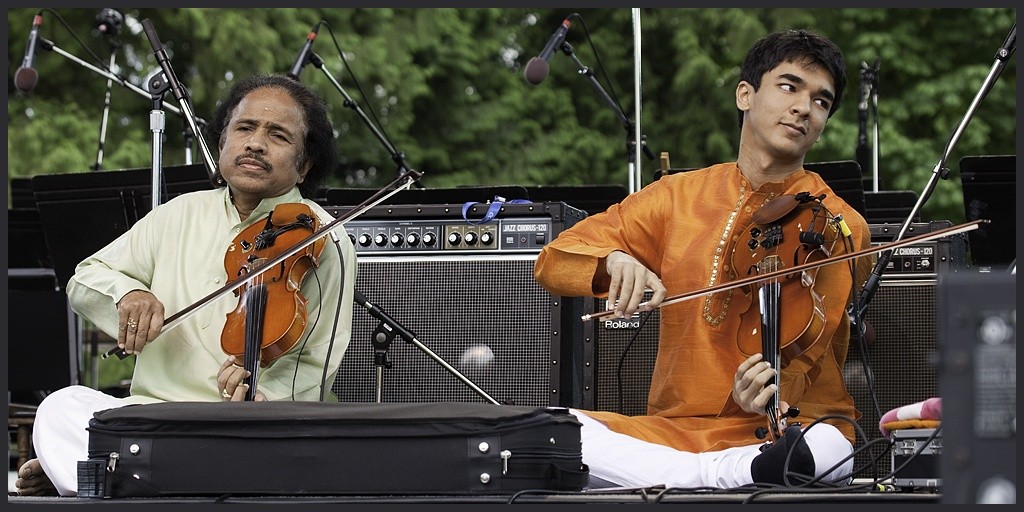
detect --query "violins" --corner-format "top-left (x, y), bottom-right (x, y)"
top-left (219, 202), bottom-right (329, 401)
top-left (730, 199), bottom-right (840, 452)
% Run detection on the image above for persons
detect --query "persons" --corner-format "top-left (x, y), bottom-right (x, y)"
top-left (533, 28), bottom-right (872, 490)
top-left (15, 74), bottom-right (359, 497)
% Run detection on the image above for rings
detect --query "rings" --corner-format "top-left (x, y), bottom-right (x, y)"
top-left (127, 317), bottom-right (138, 329)
top-left (221, 389), bottom-right (232, 401)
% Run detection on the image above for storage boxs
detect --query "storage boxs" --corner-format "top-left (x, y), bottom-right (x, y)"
top-left (889, 428), bottom-right (943, 493)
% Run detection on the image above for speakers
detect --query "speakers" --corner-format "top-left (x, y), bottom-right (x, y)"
top-left (322, 203), bottom-right (1021, 505)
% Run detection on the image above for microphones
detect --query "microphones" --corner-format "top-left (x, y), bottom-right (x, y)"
top-left (524, 14), bottom-right (574, 86)
top-left (857, 104), bottom-right (872, 174)
top-left (15, 10), bottom-right (42, 93)
top-left (287, 23), bottom-right (320, 82)
top-left (849, 320), bottom-right (875, 350)
